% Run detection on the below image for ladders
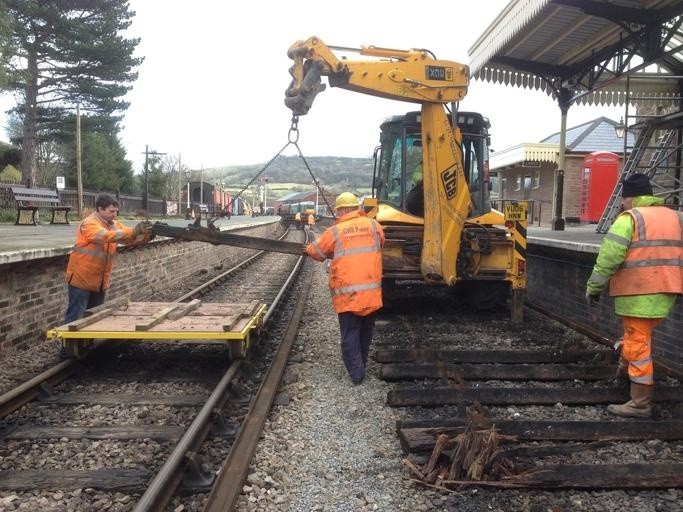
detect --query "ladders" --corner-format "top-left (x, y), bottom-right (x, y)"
top-left (644, 129), bottom-right (677, 179)
top-left (592, 117), bottom-right (654, 233)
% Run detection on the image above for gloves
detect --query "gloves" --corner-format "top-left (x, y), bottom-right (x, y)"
top-left (132, 218), bottom-right (166, 241)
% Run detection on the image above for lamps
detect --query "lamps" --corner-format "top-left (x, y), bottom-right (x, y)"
top-left (614, 116), bottom-right (639, 138)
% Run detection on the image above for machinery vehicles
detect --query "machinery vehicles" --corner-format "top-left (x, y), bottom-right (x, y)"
top-left (284, 36), bottom-right (529, 328)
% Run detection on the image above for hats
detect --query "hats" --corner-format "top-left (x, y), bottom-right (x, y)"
top-left (622, 174), bottom-right (653, 195)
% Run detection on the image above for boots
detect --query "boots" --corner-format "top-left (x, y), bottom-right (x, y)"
top-left (606, 383), bottom-right (654, 418)
top-left (614, 348), bottom-right (628, 383)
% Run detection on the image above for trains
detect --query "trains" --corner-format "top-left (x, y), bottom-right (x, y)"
top-left (277, 201), bottom-right (314, 222)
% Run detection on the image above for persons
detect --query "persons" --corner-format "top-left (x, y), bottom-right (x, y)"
top-left (582, 172), bottom-right (683, 419)
top-left (301, 189), bottom-right (387, 387)
top-left (294, 212), bottom-right (301, 230)
top-left (56, 193), bottom-right (158, 361)
top-left (306, 213), bottom-right (316, 231)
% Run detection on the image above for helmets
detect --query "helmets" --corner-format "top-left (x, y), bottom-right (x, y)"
top-left (334, 191), bottom-right (361, 209)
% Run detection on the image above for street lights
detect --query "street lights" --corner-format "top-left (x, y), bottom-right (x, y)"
top-left (184, 168), bottom-right (191, 218)
top-left (315, 177), bottom-right (319, 222)
top-left (264, 176), bottom-right (267, 216)
top-left (221, 183), bottom-right (224, 210)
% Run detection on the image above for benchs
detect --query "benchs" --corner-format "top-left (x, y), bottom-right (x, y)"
top-left (10, 184), bottom-right (72, 226)
top-left (194, 203), bottom-right (228, 220)
